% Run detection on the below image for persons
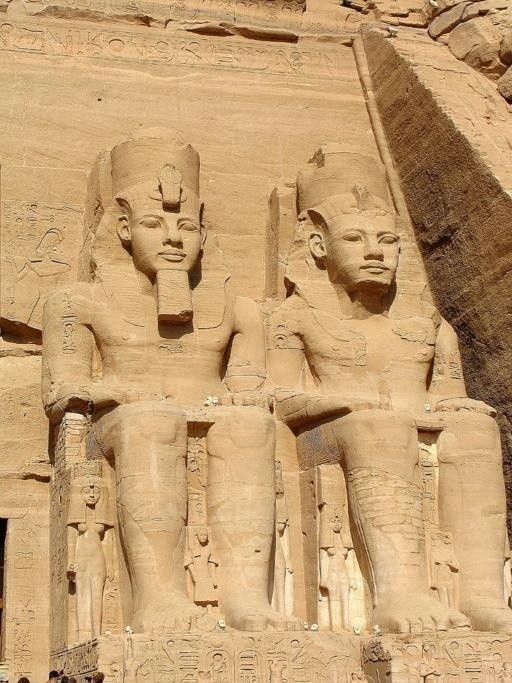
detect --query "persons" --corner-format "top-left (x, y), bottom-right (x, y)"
top-left (40, 132), bottom-right (307, 642)
top-left (263, 148), bottom-right (512, 635)
